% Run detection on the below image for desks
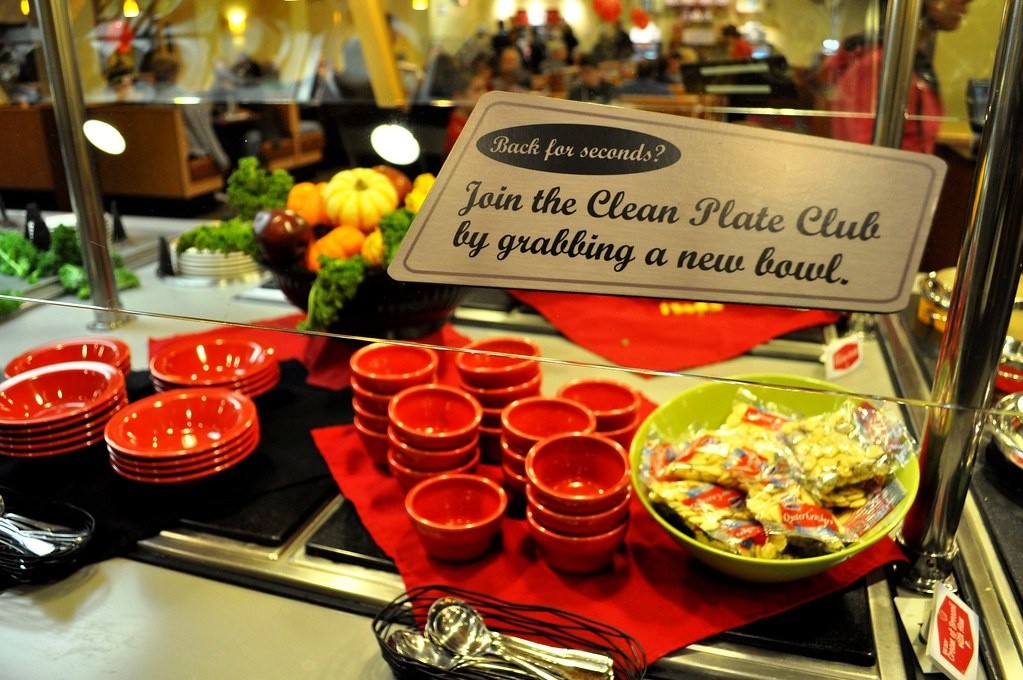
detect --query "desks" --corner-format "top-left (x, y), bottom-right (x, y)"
top-left (213, 112), bottom-right (263, 170)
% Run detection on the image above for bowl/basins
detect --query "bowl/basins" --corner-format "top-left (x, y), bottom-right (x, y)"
top-left (991, 392), bottom-right (1023, 470)
top-left (349, 334), bottom-right (641, 575)
top-left (628, 373), bottom-right (923, 584)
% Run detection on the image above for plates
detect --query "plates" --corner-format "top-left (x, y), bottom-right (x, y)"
top-left (168, 234), bottom-right (261, 279)
top-left (0, 336), bottom-right (280, 484)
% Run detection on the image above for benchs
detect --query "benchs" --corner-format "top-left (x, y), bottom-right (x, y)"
top-left (192, 92), bottom-right (324, 185)
top-left (0, 103), bottom-right (223, 216)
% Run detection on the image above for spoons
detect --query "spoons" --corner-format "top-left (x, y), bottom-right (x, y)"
top-left (385, 598), bottom-right (621, 680)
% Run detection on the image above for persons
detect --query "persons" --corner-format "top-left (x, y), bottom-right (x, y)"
top-left (454, 9), bottom-right (579, 101)
top-left (567, 54), bottom-right (624, 104)
top-left (0, 44), bottom-right (284, 169)
top-left (300, 38), bottom-right (369, 159)
top-left (721, 24), bottom-right (755, 61)
top-left (738, 34), bottom-right (940, 166)
top-left (589, 22), bottom-right (636, 62)
top-left (385, 13), bottom-right (459, 130)
top-left (442, 67), bottom-right (487, 156)
top-left (619, 50), bottom-right (682, 98)
